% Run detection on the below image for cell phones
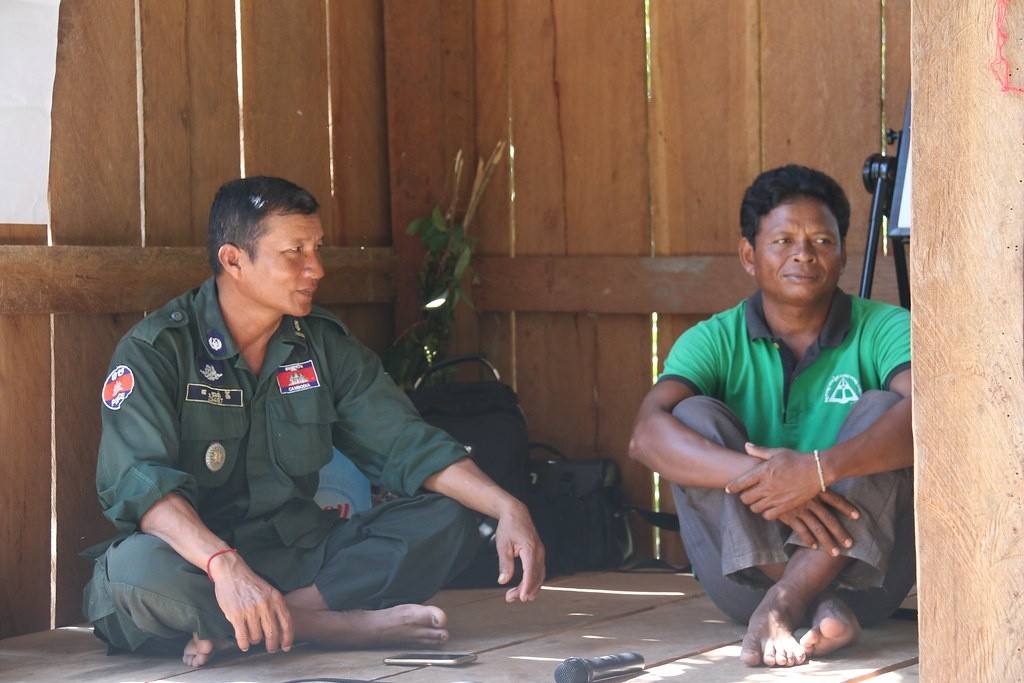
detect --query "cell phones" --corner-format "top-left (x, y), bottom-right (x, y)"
top-left (384, 652), bottom-right (477, 667)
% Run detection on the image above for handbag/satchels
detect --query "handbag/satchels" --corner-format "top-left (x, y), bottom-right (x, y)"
top-left (405, 355), bottom-right (528, 486)
top-left (499, 443), bottom-right (633, 571)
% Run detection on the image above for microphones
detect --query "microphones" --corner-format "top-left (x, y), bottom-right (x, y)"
top-left (553, 653), bottom-right (646, 683)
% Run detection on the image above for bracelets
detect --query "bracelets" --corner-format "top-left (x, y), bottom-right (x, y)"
top-left (814, 450), bottom-right (826, 492)
top-left (206, 549), bottom-right (239, 583)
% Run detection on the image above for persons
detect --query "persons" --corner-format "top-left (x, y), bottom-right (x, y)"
top-left (629, 163), bottom-right (914, 666)
top-left (82, 177), bottom-right (546, 667)
